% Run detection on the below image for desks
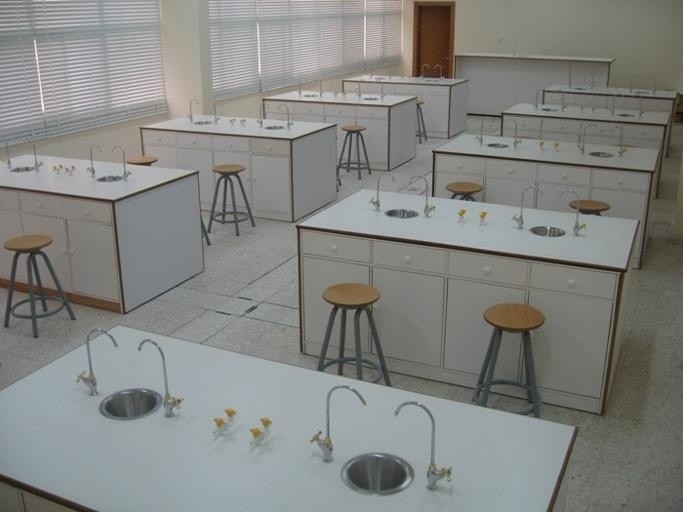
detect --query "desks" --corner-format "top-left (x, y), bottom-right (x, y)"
top-left (296, 189), bottom-right (640, 417)
top-left (431, 134), bottom-right (660, 272)
top-left (539, 83), bottom-right (678, 158)
top-left (498, 102), bottom-right (670, 200)
top-left (342, 73), bottom-right (470, 139)
top-left (138, 114), bottom-right (338, 223)
top-left (263, 90), bottom-right (419, 172)
top-left (2, 153), bottom-right (205, 314)
top-left (1, 324), bottom-right (579, 511)
top-left (456, 55), bottom-right (616, 118)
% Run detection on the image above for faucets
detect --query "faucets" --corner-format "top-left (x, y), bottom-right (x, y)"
top-left (179, 61), bottom-right (661, 164)
top-left (309, 385), bottom-right (368, 464)
top-left (408, 175), bottom-right (433, 218)
top-left (112, 144), bottom-right (132, 181)
top-left (86, 143), bottom-right (102, 179)
top-left (369, 172), bottom-right (395, 212)
top-left (573, 193), bottom-right (585, 236)
top-left (32, 141), bottom-right (44, 172)
top-left (395, 401), bottom-right (452, 491)
top-left (512, 186), bottom-right (538, 229)
top-left (76, 328), bottom-right (120, 396)
top-left (137, 338), bottom-right (185, 416)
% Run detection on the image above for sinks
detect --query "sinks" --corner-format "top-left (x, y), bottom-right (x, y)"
top-left (99, 387), bottom-right (163, 419)
top-left (385, 209), bottom-right (419, 220)
top-left (529, 225), bottom-right (565, 238)
top-left (340, 452), bottom-right (416, 498)
top-left (10, 166), bottom-right (34, 173)
top-left (97, 175), bottom-right (122, 182)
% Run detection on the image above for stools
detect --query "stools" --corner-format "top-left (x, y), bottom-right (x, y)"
top-left (4, 235), bottom-right (77, 338)
top-left (471, 303), bottom-right (546, 422)
top-left (336, 126), bottom-right (373, 180)
top-left (208, 164), bottom-right (257, 235)
top-left (417, 99), bottom-right (428, 145)
top-left (199, 216), bottom-right (212, 247)
top-left (315, 281), bottom-right (391, 387)
